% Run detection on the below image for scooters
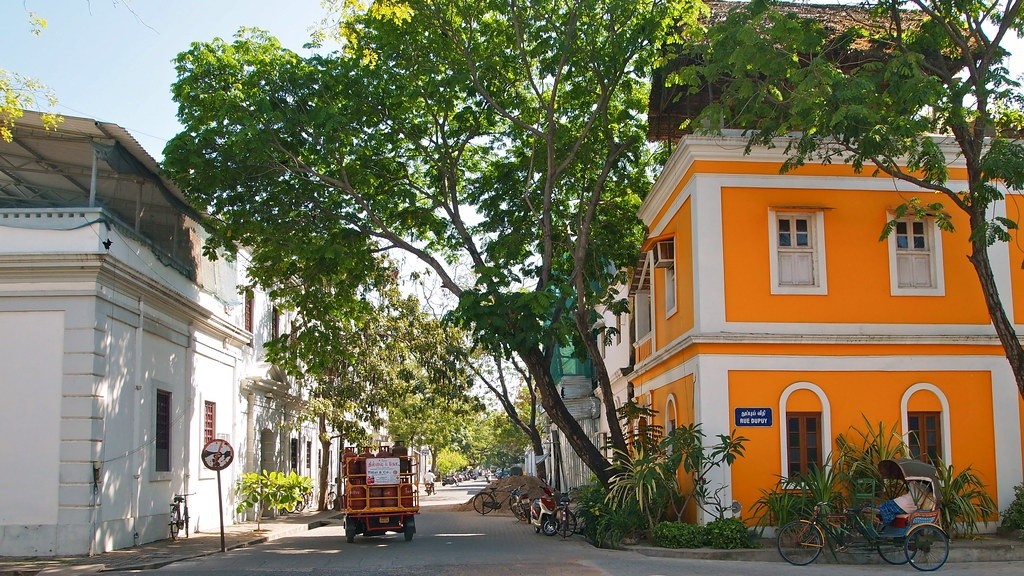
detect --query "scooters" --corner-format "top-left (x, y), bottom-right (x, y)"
top-left (443, 475), bottom-right (459, 486)
top-left (530, 486), bottom-right (562, 536)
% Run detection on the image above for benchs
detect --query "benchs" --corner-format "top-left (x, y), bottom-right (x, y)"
top-left (891, 493), bottom-right (935, 528)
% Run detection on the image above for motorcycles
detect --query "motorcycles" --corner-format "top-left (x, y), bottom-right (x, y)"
top-left (508, 483), bottom-right (531, 523)
top-left (424, 481), bottom-right (433, 496)
top-left (343, 446), bottom-right (420, 541)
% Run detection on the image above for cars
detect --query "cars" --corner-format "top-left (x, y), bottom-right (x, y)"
top-left (455, 471), bottom-right (478, 481)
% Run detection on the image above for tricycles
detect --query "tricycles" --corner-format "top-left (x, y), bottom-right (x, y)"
top-left (777, 458), bottom-right (951, 572)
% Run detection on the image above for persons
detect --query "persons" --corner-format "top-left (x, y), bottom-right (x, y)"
top-left (424, 470), bottom-right (438, 495)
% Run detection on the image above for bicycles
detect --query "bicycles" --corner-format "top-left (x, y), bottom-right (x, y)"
top-left (290, 485), bottom-right (338, 512)
top-left (473, 484), bottom-right (526, 515)
top-left (169, 493), bottom-right (195, 540)
top-left (551, 495), bottom-right (578, 539)
